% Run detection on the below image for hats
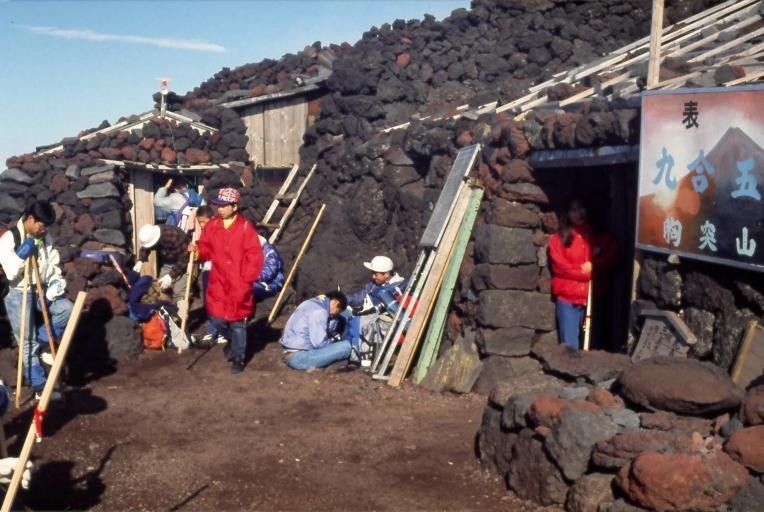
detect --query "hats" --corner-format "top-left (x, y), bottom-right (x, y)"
top-left (211, 187), bottom-right (241, 205)
top-left (138, 224), bottom-right (161, 248)
top-left (363, 256), bottom-right (394, 273)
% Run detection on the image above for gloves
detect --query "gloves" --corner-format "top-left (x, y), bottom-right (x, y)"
top-left (133, 260), bottom-right (143, 272)
top-left (158, 274), bottom-right (172, 289)
top-left (38, 295), bottom-right (52, 309)
top-left (17, 237), bottom-right (37, 260)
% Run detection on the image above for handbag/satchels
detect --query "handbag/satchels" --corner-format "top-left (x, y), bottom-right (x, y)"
top-left (165, 212), bottom-right (183, 225)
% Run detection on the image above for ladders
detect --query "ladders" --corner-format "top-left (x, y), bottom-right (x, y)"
top-left (255, 161), bottom-right (317, 246)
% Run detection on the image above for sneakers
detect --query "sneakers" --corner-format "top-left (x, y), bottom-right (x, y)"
top-left (33, 342), bottom-right (65, 368)
top-left (35, 391), bottom-right (64, 401)
top-left (203, 333), bottom-right (244, 373)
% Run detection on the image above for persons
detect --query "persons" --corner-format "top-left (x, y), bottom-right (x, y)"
top-left (0, 456), bottom-right (33, 492)
top-left (546, 191), bottom-right (617, 353)
top-left (273, 283), bottom-right (357, 376)
top-left (0, 197), bottom-right (88, 408)
top-left (340, 252), bottom-right (421, 372)
top-left (119, 169), bottom-right (287, 376)
